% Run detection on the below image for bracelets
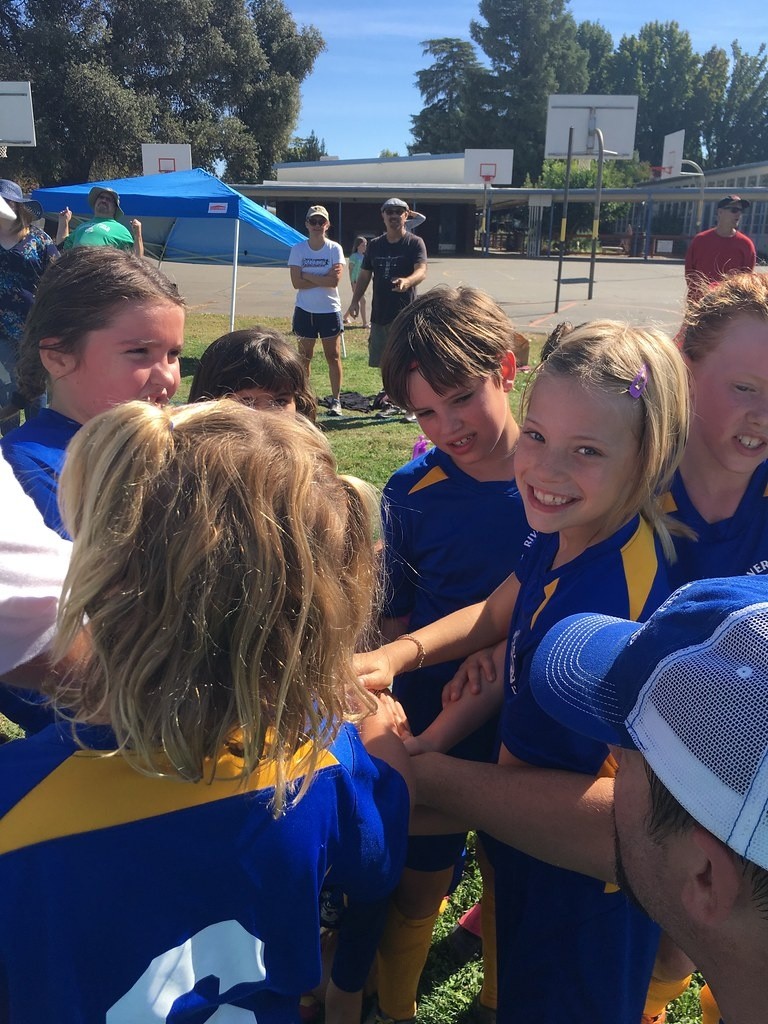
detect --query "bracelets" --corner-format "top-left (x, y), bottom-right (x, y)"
top-left (395, 634), bottom-right (426, 672)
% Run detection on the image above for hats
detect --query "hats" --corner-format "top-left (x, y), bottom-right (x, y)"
top-left (530, 574), bottom-right (766, 871)
top-left (718, 195), bottom-right (750, 209)
top-left (382, 197), bottom-right (409, 212)
top-left (88, 186), bottom-right (124, 220)
top-left (0, 179), bottom-right (43, 221)
top-left (306, 205), bottom-right (329, 223)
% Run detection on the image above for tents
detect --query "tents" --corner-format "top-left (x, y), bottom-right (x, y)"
top-left (31, 168), bottom-right (312, 335)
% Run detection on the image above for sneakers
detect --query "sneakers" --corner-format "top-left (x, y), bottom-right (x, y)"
top-left (376, 400), bottom-right (399, 418)
top-left (326, 398), bottom-right (342, 416)
top-left (400, 410), bottom-right (417, 424)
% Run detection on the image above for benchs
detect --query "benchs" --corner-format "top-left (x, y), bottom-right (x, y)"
top-left (601, 246), bottom-right (623, 256)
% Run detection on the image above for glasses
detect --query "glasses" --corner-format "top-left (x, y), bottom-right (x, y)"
top-left (98, 194), bottom-right (114, 202)
top-left (384, 208), bottom-right (405, 215)
top-left (307, 218), bottom-right (326, 226)
top-left (723, 206), bottom-right (744, 215)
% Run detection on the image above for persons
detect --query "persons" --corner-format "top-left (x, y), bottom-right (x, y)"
top-left (343, 236), bottom-right (372, 328)
top-left (341, 322), bottom-right (768, 1024)
top-left (0, 247), bottom-right (185, 739)
top-left (365, 287), bottom-right (544, 1024)
top-left (186, 326), bottom-right (315, 426)
top-left (0, 178), bottom-right (63, 436)
top-left (1, 398), bottom-right (416, 1024)
top-left (352, 320), bottom-right (691, 1024)
top-left (350, 198), bottom-right (427, 423)
top-left (54, 186), bottom-right (144, 257)
top-left (685, 195), bottom-right (756, 302)
top-left (287, 205), bottom-right (346, 416)
top-left (0, 446), bottom-right (102, 700)
top-left (410, 575), bottom-right (768, 1024)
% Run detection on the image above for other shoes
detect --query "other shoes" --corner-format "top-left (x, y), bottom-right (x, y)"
top-left (299, 992), bottom-right (320, 1023)
top-left (454, 989), bottom-right (497, 1024)
top-left (448, 895), bottom-right (486, 954)
top-left (343, 318), bottom-right (351, 324)
top-left (362, 323), bottom-right (370, 328)
